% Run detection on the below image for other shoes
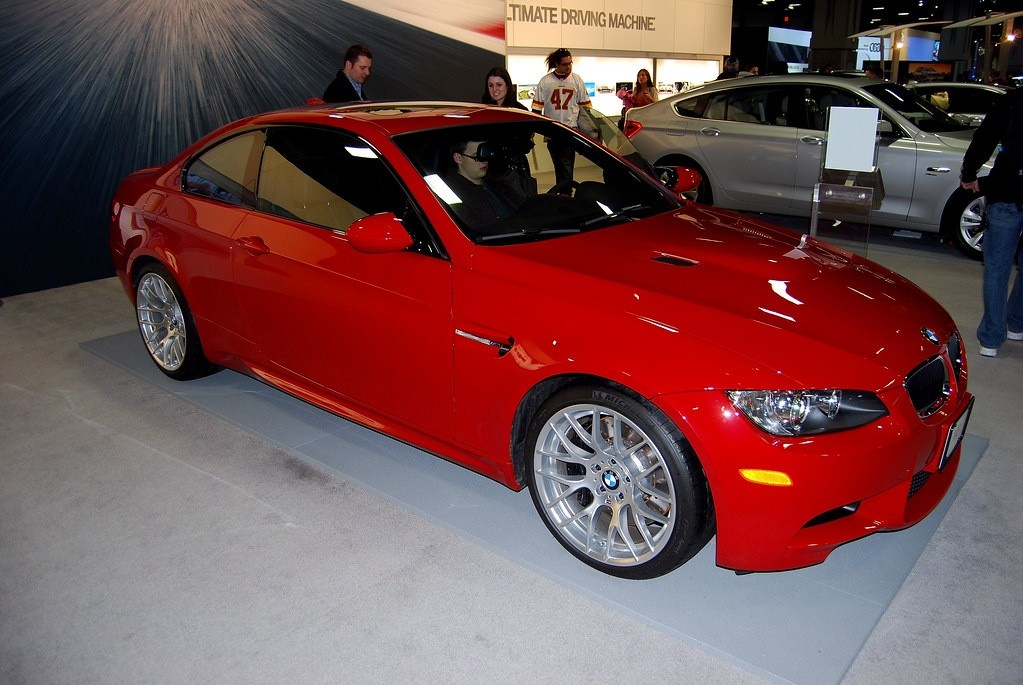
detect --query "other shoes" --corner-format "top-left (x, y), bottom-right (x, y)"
top-left (980, 328), bottom-right (1023, 357)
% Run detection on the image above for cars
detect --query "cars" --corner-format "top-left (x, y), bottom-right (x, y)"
top-left (621, 70), bottom-right (1002, 261)
top-left (105, 104), bottom-right (971, 577)
top-left (901, 78), bottom-right (1008, 131)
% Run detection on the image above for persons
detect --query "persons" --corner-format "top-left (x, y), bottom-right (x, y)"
top-left (620, 69), bottom-right (658, 112)
top-left (322, 45), bottom-right (374, 108)
top-left (958, 82), bottom-right (1022, 357)
top-left (531, 48), bottom-right (596, 197)
top-left (984, 69), bottom-right (1023, 91)
top-left (739, 64), bottom-right (758, 77)
top-left (470, 67), bottom-right (534, 182)
top-left (862, 63), bottom-right (884, 81)
top-left (445, 134), bottom-right (568, 238)
top-left (715, 56), bottom-right (739, 80)
top-left (810, 63), bottom-right (836, 130)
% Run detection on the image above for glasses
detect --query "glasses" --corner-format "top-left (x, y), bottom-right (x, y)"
top-left (557, 61), bottom-right (574, 67)
